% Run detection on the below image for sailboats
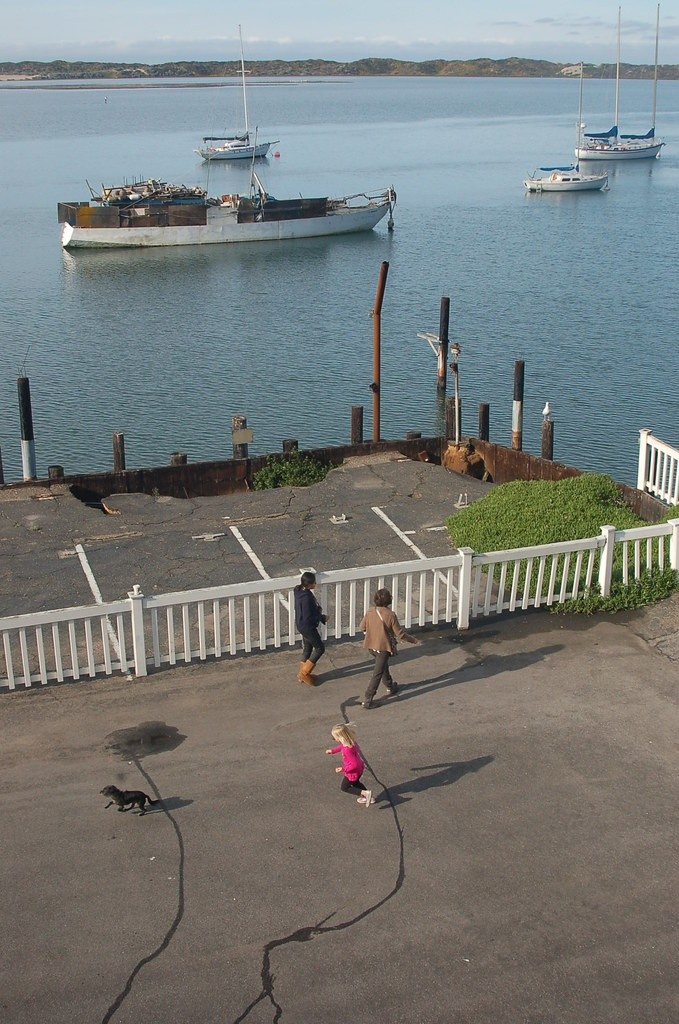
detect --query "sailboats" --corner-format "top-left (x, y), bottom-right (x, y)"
top-left (523, 63), bottom-right (610, 192)
top-left (574, 3), bottom-right (666, 160)
top-left (190, 25), bottom-right (281, 160)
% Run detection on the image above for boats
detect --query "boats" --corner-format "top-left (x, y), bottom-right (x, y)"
top-left (54, 179), bottom-right (402, 248)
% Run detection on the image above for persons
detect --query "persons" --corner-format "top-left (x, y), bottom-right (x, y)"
top-left (357, 589), bottom-right (419, 710)
top-left (325, 724), bottom-right (377, 807)
top-left (293, 572), bottom-right (329, 688)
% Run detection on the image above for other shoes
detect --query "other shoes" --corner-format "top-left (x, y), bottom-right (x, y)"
top-left (366, 790), bottom-right (372, 807)
top-left (356, 796), bottom-right (377, 804)
top-left (387, 681), bottom-right (397, 692)
top-left (362, 702), bottom-right (374, 709)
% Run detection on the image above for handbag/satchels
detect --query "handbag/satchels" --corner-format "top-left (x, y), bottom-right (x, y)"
top-left (390, 635), bottom-right (399, 657)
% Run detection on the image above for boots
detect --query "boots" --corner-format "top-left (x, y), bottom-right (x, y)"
top-left (298, 661), bottom-right (317, 683)
top-left (297, 659), bottom-right (316, 686)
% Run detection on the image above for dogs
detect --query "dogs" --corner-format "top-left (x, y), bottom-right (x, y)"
top-left (100, 785), bottom-right (159, 816)
